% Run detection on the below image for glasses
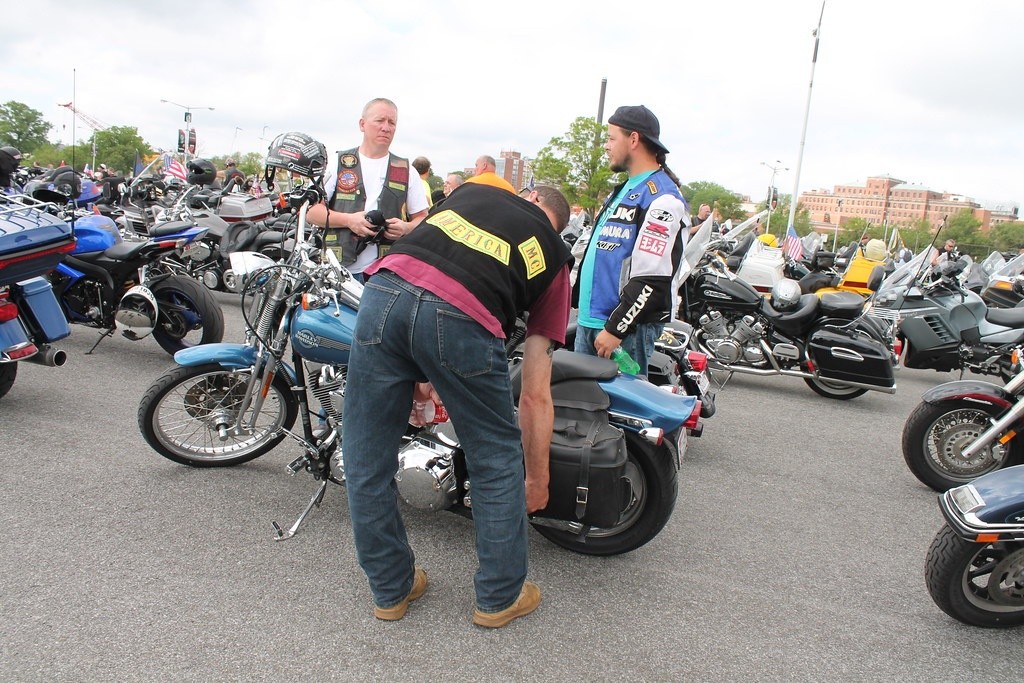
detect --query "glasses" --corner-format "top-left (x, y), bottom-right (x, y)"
top-left (948, 244), bottom-right (953, 247)
top-left (519, 188), bottom-right (540, 202)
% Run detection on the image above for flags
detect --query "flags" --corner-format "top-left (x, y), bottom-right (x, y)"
top-left (165, 154), bottom-right (189, 180)
top-left (784, 226), bottom-right (803, 262)
top-left (135, 152), bottom-right (145, 177)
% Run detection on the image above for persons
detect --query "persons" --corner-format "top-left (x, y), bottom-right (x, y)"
top-left (573, 105), bottom-right (687, 380)
top-left (402, 156), bottom-right (434, 221)
top-left (932, 239), bottom-right (955, 265)
top-left (859, 236), bottom-right (869, 254)
top-left (216, 158), bottom-right (243, 190)
top-left (465, 155), bottom-right (517, 195)
top-left (95, 163), bottom-right (110, 180)
top-left (33, 161), bottom-right (40, 166)
top-left (294, 97), bottom-right (427, 286)
top-left (342, 180), bottom-right (578, 624)
top-left (0, 147), bottom-right (23, 188)
top-left (687, 203), bottom-right (710, 242)
top-left (431, 174), bottom-right (464, 207)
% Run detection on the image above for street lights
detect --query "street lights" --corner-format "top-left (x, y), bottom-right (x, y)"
top-left (762, 159), bottom-right (791, 235)
top-left (161, 100), bottom-right (216, 167)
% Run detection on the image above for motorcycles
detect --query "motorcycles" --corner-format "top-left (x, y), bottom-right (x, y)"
top-left (0, 161), bottom-right (1024, 496)
top-left (671, 209), bottom-right (898, 399)
top-left (136, 174), bottom-right (704, 558)
top-left (924, 464), bottom-right (1024, 629)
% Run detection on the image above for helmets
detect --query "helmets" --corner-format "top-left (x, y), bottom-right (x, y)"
top-left (186, 159), bottom-right (216, 185)
top-left (224, 158), bottom-right (236, 168)
top-left (54, 172), bottom-right (82, 199)
top-left (759, 234), bottom-right (778, 248)
top-left (771, 279), bottom-right (801, 312)
top-left (266, 131), bottom-right (328, 177)
top-left (0, 146), bottom-right (22, 171)
top-left (114, 286), bottom-right (159, 341)
top-left (100, 164), bottom-right (106, 170)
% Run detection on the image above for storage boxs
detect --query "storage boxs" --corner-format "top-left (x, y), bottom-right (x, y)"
top-left (808, 326), bottom-right (895, 387)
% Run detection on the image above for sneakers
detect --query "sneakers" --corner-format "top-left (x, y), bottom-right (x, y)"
top-left (374, 569), bottom-right (428, 620)
top-left (474, 582), bottom-right (541, 628)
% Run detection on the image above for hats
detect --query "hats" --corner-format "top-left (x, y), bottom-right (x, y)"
top-left (608, 105), bottom-right (669, 153)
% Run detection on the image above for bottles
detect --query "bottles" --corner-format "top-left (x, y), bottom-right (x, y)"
top-left (409, 399), bottom-right (451, 423)
top-left (596, 333), bottom-right (640, 375)
